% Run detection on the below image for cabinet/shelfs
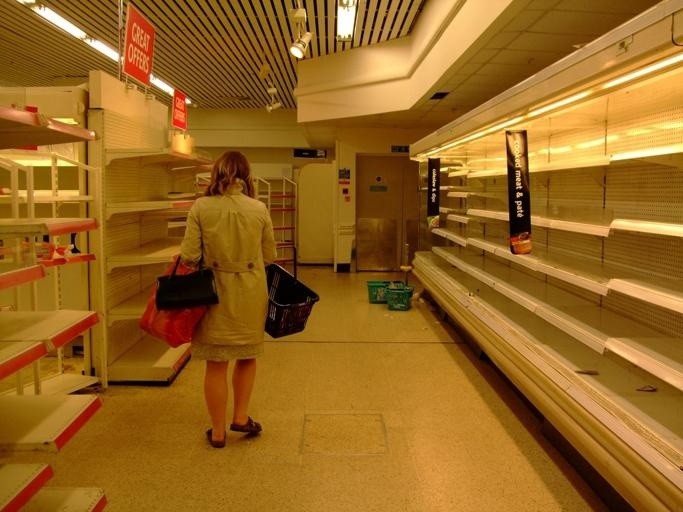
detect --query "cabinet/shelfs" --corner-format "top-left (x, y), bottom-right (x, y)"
top-left (407, 1), bottom-right (683, 512)
top-left (168, 153), bottom-right (212, 236)
top-left (1, 106), bottom-right (108, 512)
top-left (252, 175), bottom-right (296, 264)
top-left (1, 88), bottom-right (92, 385)
top-left (88, 69), bottom-right (213, 388)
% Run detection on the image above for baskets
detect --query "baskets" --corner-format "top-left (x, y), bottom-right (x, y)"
top-left (265, 263), bottom-right (319, 338)
top-left (366, 280), bottom-right (414, 311)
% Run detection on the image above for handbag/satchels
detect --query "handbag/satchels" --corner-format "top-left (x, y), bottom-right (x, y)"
top-left (156, 269), bottom-right (218, 309)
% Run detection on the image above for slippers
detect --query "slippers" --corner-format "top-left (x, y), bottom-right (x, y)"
top-left (230, 417), bottom-right (262, 432)
top-left (207, 428), bottom-right (226, 448)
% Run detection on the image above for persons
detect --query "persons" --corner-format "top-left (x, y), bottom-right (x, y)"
top-left (175, 150), bottom-right (278, 448)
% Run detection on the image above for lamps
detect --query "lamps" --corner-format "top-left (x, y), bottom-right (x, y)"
top-left (289, 8), bottom-right (313, 59)
